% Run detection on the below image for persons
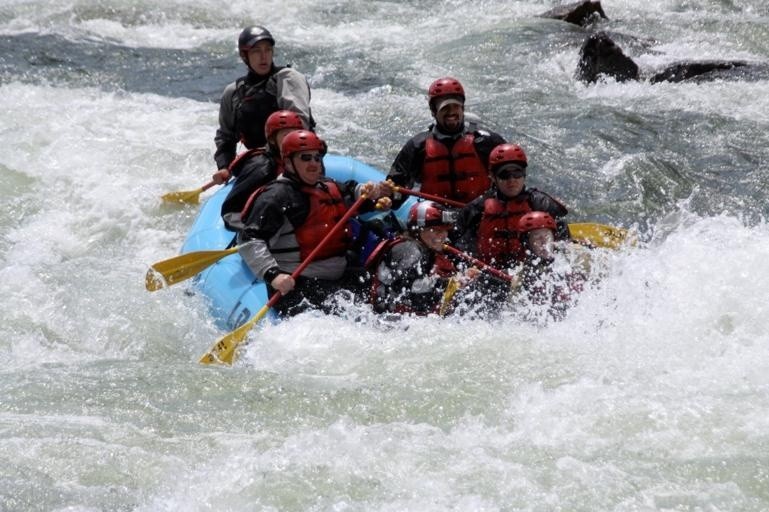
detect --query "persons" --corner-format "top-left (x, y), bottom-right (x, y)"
top-left (377, 77), bottom-right (510, 218)
top-left (222, 111), bottom-right (310, 234)
top-left (518, 211), bottom-right (588, 320)
top-left (214, 26), bottom-right (317, 185)
top-left (370, 199), bottom-right (506, 320)
top-left (239, 131), bottom-right (368, 321)
top-left (449, 143), bottom-right (574, 271)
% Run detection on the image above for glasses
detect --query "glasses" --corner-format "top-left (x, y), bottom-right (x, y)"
top-left (496, 169), bottom-right (524, 180)
top-left (294, 154), bottom-right (322, 161)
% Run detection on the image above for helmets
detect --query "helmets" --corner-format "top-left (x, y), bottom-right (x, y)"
top-left (408, 200), bottom-right (454, 230)
top-left (518, 211), bottom-right (556, 234)
top-left (488, 143), bottom-right (527, 173)
top-left (429, 78), bottom-right (465, 112)
top-left (239, 26), bottom-right (275, 51)
top-left (265, 110), bottom-right (324, 158)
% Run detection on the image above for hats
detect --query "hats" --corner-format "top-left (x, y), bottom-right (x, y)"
top-left (493, 163), bottom-right (523, 174)
top-left (435, 100), bottom-right (463, 112)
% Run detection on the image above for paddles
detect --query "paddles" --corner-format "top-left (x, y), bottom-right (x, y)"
top-left (387, 177), bottom-right (638, 253)
top-left (145, 196), bottom-right (387, 292)
top-left (159, 168), bottom-right (229, 206)
top-left (199, 180), bottom-right (373, 367)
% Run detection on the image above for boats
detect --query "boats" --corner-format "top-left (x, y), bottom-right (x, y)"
top-left (179, 153), bottom-right (521, 380)
